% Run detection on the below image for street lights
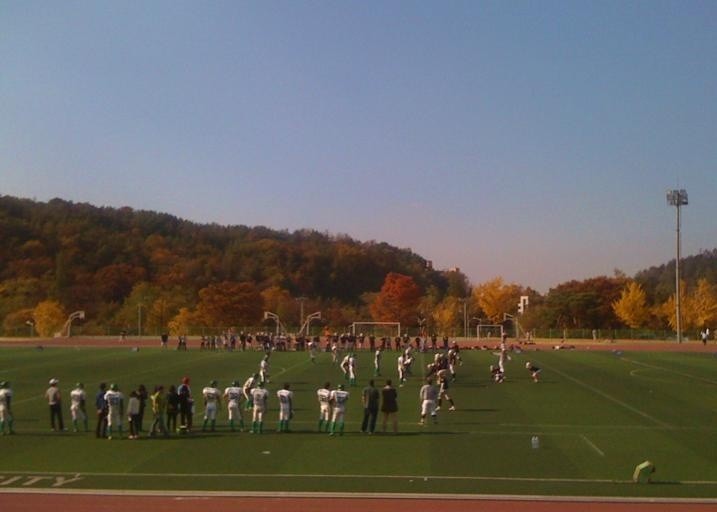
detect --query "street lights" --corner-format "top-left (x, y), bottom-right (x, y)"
top-left (665, 188), bottom-right (687, 344)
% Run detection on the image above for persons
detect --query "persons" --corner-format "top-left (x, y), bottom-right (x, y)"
top-left (249, 381), bottom-right (268, 434)
top-left (0, 381), bottom-right (15, 435)
top-left (359, 379), bottom-right (378, 434)
top-left (526, 362), bottom-right (543, 383)
top-left (45, 378), bottom-right (67, 431)
top-left (381, 379), bottom-right (401, 435)
top-left (417, 376), bottom-right (438, 424)
top-left (526, 330), bottom-right (531, 340)
top-left (242, 373), bottom-right (258, 411)
top-left (502, 331), bottom-right (508, 343)
top-left (309, 341), bottom-right (463, 411)
top-left (275, 382), bottom-right (293, 432)
top-left (69, 382), bottom-right (89, 432)
top-left (552, 345), bottom-right (573, 350)
top-left (200, 332), bottom-right (459, 351)
top-left (329, 384), bottom-right (349, 435)
top-left (316, 382), bottom-right (330, 433)
top-left (592, 329), bottom-right (595, 340)
top-left (224, 379), bottom-right (243, 431)
top-left (258, 354), bottom-right (268, 388)
top-left (95, 376), bottom-right (193, 440)
top-left (174, 333), bottom-right (186, 352)
top-left (160, 331), bottom-right (169, 352)
top-left (559, 336), bottom-right (564, 345)
top-left (489, 363), bottom-right (507, 384)
top-left (202, 380), bottom-right (220, 432)
top-left (700, 329), bottom-right (708, 345)
top-left (491, 344), bottom-right (511, 373)
